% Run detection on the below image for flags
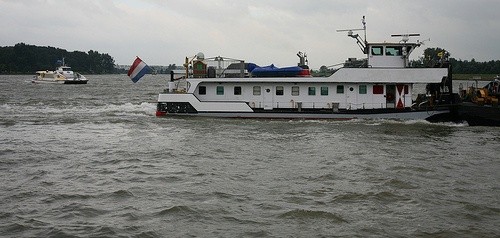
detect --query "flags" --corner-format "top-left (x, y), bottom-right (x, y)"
top-left (128, 57), bottom-right (152, 84)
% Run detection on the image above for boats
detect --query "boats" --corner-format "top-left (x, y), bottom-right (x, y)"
top-left (32, 57), bottom-right (88, 83)
top-left (453, 79), bottom-right (500, 126)
top-left (154, 16), bottom-right (457, 119)
